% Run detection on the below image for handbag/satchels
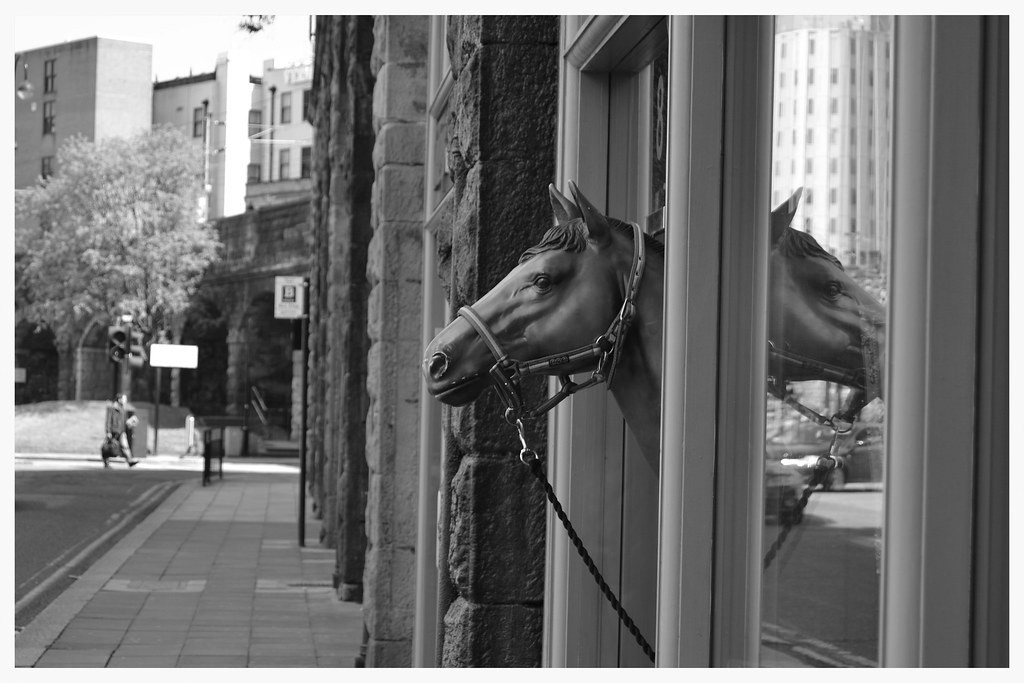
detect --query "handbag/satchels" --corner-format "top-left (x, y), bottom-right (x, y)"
top-left (102, 438), bottom-right (122, 459)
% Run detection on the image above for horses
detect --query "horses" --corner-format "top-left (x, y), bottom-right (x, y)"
top-left (768, 185), bottom-right (888, 406)
top-left (422, 180), bottom-right (641, 408)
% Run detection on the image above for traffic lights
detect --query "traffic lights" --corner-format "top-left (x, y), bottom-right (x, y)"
top-left (128, 328), bottom-right (146, 370)
top-left (107, 326), bottom-right (127, 365)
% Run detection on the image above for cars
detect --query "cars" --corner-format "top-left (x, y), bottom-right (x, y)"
top-left (765, 415), bottom-right (884, 495)
top-left (763, 459), bottom-right (806, 524)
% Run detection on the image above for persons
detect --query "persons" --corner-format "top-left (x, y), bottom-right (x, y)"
top-left (101, 393), bottom-right (139, 468)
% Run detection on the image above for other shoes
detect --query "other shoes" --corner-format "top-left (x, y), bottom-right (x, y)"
top-left (129, 462), bottom-right (138, 467)
top-left (103, 457), bottom-right (109, 468)
